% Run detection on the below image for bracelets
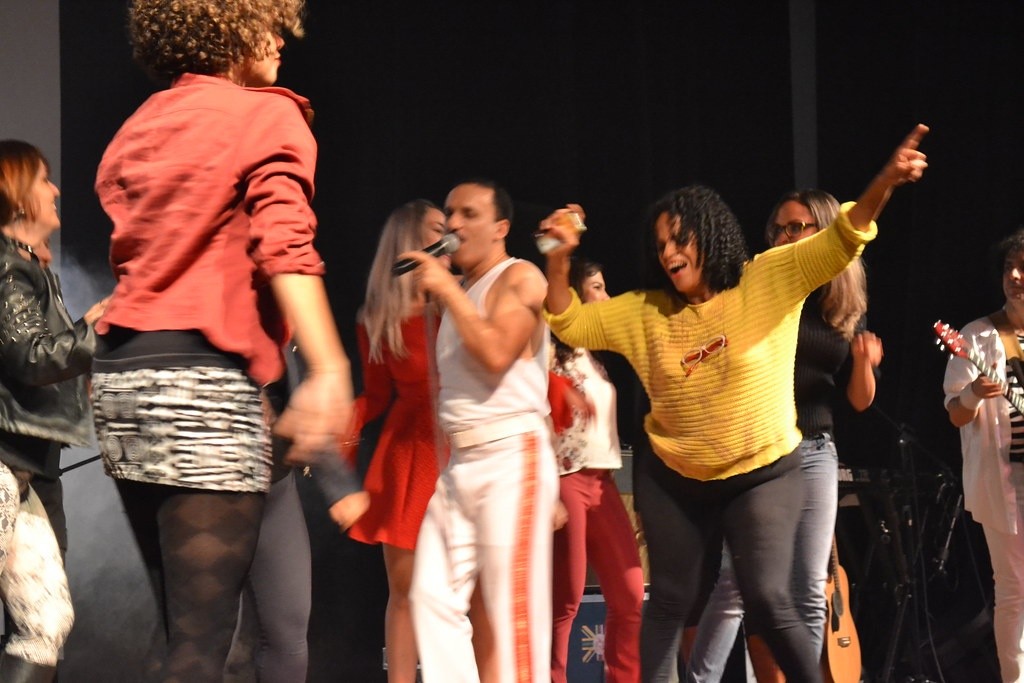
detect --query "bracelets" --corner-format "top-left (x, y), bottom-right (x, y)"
top-left (959, 383), bottom-right (984, 410)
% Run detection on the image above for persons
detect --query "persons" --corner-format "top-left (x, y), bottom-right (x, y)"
top-left (140, 123), bottom-right (927, 683)
top-left (0, 138), bottom-right (114, 683)
top-left (942, 234), bottom-right (1024, 683)
top-left (90, 0), bottom-right (354, 683)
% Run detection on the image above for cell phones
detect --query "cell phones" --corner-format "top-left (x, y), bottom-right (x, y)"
top-left (535, 213), bottom-right (586, 253)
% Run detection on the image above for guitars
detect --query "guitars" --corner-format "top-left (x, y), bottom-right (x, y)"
top-left (821, 530), bottom-right (864, 683)
top-left (929, 317), bottom-right (1024, 419)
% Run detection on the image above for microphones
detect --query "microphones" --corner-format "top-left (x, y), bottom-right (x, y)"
top-left (389, 233), bottom-right (460, 277)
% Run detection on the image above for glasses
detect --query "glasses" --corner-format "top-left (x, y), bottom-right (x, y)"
top-left (767, 219), bottom-right (818, 240)
